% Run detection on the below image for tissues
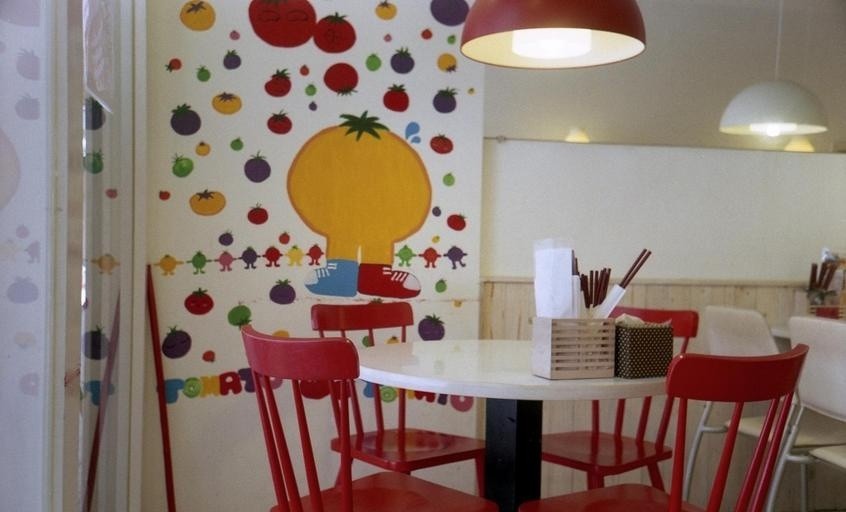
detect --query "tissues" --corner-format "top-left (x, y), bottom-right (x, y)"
top-left (614, 313), bottom-right (674, 379)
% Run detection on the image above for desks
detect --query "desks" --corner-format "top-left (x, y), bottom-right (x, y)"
top-left (344, 336), bottom-right (667, 511)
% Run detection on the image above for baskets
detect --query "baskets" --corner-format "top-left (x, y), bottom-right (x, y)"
top-left (531, 317), bottom-right (615, 380)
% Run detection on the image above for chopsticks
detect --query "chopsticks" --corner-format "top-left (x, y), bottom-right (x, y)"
top-left (576, 269), bottom-right (610, 311)
top-left (807, 259), bottom-right (838, 293)
top-left (620, 248), bottom-right (652, 287)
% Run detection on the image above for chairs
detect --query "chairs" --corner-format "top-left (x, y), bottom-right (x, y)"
top-left (680, 303), bottom-right (845, 512)
top-left (530, 302), bottom-right (703, 494)
top-left (231, 320), bottom-right (499, 512)
top-left (300, 299), bottom-right (484, 498)
top-left (766, 310), bottom-right (846, 510)
top-left (516, 342), bottom-right (807, 511)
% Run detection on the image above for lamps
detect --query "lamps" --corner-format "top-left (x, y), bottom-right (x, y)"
top-left (457, 0), bottom-right (652, 74)
top-left (717, 0), bottom-right (833, 137)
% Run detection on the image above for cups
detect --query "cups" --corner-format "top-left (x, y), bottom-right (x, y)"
top-left (816, 307), bottom-right (839, 320)
